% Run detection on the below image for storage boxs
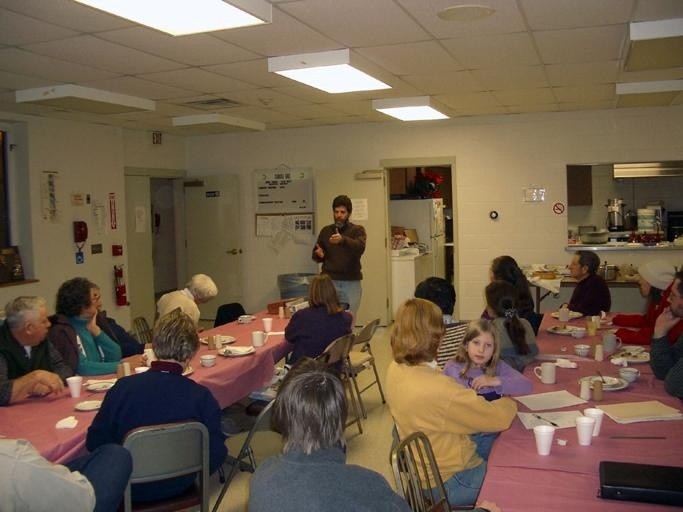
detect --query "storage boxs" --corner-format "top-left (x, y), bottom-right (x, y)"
top-left (392, 229), bottom-right (417, 245)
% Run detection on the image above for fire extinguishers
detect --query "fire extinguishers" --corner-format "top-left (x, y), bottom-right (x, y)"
top-left (114, 263), bottom-right (127, 305)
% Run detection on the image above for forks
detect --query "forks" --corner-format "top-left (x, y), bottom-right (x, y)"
top-left (597, 370), bottom-right (606, 384)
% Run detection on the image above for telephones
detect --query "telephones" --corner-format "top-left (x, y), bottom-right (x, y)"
top-left (72, 221), bottom-right (89, 243)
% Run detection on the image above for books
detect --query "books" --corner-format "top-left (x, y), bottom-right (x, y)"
top-left (596, 401), bottom-right (682, 425)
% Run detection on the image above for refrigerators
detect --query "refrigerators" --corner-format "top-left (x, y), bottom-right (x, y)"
top-left (389, 198), bottom-right (446, 284)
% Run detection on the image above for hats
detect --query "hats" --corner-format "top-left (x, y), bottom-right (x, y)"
top-left (637, 259), bottom-right (676, 291)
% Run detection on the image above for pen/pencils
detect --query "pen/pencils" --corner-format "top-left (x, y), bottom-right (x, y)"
top-left (532, 413), bottom-right (559, 427)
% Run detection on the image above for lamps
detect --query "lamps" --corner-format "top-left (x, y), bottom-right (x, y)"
top-left (172, 114), bottom-right (266, 131)
top-left (73, 0), bottom-right (273, 37)
top-left (16, 83), bottom-right (157, 115)
top-left (371, 96), bottom-right (452, 121)
top-left (613, 80), bottom-right (683, 107)
top-left (267, 48), bottom-right (394, 94)
top-left (623, 18), bottom-right (683, 71)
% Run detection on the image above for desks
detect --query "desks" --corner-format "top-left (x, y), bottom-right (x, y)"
top-left (526, 269), bottom-right (640, 313)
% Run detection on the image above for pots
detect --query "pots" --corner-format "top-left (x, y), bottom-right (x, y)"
top-left (598, 261), bottom-right (617, 281)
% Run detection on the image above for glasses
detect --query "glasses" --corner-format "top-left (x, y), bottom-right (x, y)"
top-left (669, 292), bottom-right (681, 301)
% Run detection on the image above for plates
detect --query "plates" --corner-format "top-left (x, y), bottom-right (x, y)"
top-left (181, 367), bottom-right (193, 377)
top-left (551, 312), bottom-right (583, 317)
top-left (576, 374), bottom-right (629, 390)
top-left (199, 336), bottom-right (236, 344)
top-left (237, 315), bottom-right (256, 323)
top-left (620, 352), bottom-right (650, 362)
top-left (75, 400), bottom-right (103, 411)
top-left (87, 381), bottom-right (114, 392)
top-left (547, 325), bottom-right (578, 335)
top-left (218, 346), bottom-right (256, 356)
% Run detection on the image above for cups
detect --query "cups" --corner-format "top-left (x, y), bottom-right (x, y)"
top-left (559, 309), bottom-right (568, 321)
top-left (66, 376), bottom-right (82, 397)
top-left (534, 363), bottom-right (559, 384)
top-left (134, 366), bottom-right (150, 373)
top-left (571, 328), bottom-right (585, 338)
top-left (603, 334), bottom-right (621, 352)
top-left (262, 318), bottom-right (273, 332)
top-left (584, 408), bottom-right (603, 437)
top-left (619, 368), bottom-right (640, 382)
top-left (143, 349), bottom-right (158, 366)
top-left (533, 425), bottom-right (555, 456)
top-left (576, 417), bottom-right (595, 446)
top-left (252, 331), bottom-right (268, 347)
top-left (199, 355), bottom-right (216, 367)
top-left (574, 344), bottom-right (590, 357)
top-left (586, 316), bottom-right (596, 336)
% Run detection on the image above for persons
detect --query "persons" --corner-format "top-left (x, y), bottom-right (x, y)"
top-left (156, 272), bottom-right (219, 333)
top-left (286, 273), bottom-right (354, 373)
top-left (312, 195), bottom-right (366, 329)
top-left (415, 276), bottom-right (474, 371)
top-left (560, 250), bottom-right (611, 315)
top-left (94, 284), bottom-right (153, 357)
top-left (382, 298), bottom-right (518, 508)
top-left (486, 281), bottom-right (538, 372)
top-left (85, 309), bottom-right (227, 502)
top-left (249, 370), bottom-right (502, 511)
top-left (1, 439), bottom-right (133, 511)
top-left (480, 256), bottom-right (544, 335)
top-left (47, 277), bottom-right (145, 387)
top-left (600, 261), bottom-right (683, 347)
top-left (442, 318), bottom-right (534, 460)
top-left (0, 296), bottom-right (76, 406)
top-left (649, 272), bottom-right (683, 399)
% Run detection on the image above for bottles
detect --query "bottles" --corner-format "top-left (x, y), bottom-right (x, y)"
top-left (215, 334), bottom-right (222, 348)
top-left (580, 380), bottom-right (591, 400)
top-left (591, 315), bottom-right (600, 328)
top-left (116, 365), bottom-right (124, 378)
top-left (208, 336), bottom-right (216, 349)
top-left (594, 344), bottom-right (604, 360)
top-left (593, 381), bottom-right (602, 400)
top-left (123, 362), bottom-right (130, 375)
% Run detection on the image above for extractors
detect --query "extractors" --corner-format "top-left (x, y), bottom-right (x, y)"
top-left (613, 161), bottom-right (683, 182)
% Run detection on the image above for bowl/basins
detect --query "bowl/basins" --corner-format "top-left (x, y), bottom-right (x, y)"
top-left (580, 232), bottom-right (608, 243)
top-left (591, 377), bottom-right (619, 388)
top-left (623, 346), bottom-right (645, 356)
